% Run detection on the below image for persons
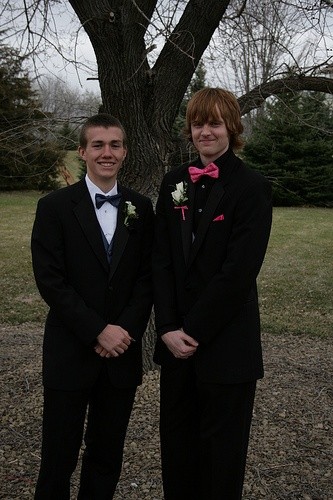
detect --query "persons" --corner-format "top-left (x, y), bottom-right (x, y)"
top-left (151, 85), bottom-right (274, 500)
top-left (30, 114), bottom-right (157, 500)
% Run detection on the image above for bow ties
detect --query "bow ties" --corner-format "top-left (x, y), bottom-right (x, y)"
top-left (94, 192), bottom-right (121, 210)
top-left (188, 162), bottom-right (219, 183)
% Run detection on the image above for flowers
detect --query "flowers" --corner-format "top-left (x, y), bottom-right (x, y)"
top-left (123, 200), bottom-right (138, 227)
top-left (171, 181), bottom-right (190, 221)
